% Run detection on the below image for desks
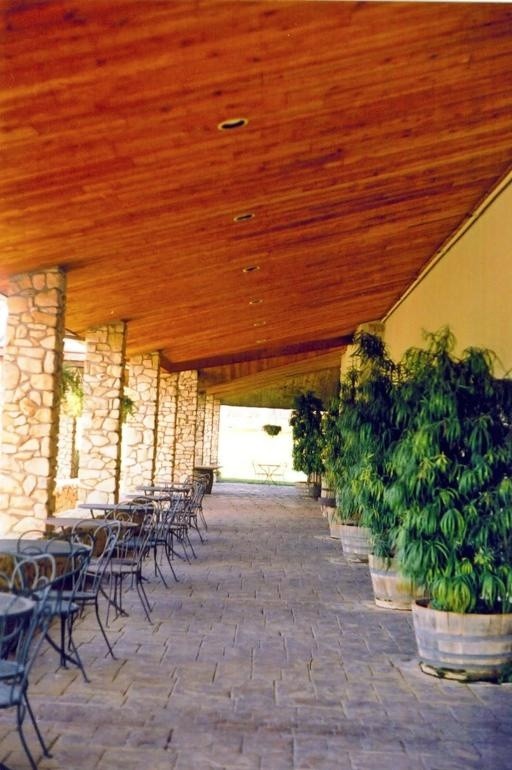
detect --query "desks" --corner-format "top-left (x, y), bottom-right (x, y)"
top-left (0, 591), bottom-right (34, 618)
top-left (44, 518), bottom-right (139, 617)
top-left (0, 539), bottom-right (92, 667)
top-left (78, 487), bottom-right (189, 512)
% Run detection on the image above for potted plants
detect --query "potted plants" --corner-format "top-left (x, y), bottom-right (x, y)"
top-left (320, 326), bottom-right (511, 682)
top-left (291, 389), bottom-right (325, 500)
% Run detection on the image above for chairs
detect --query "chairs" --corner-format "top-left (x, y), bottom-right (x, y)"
top-left (18, 530), bottom-right (95, 667)
top-left (117, 475), bottom-right (210, 588)
top-left (0, 591), bottom-right (49, 684)
top-left (83, 514), bottom-right (157, 625)
top-left (0, 552), bottom-right (56, 614)
top-left (0, 605), bottom-right (52, 770)
